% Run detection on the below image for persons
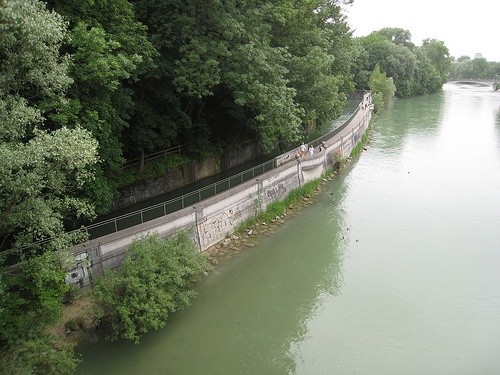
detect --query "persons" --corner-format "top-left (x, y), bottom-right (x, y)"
top-left (360, 101), bottom-right (370, 109)
top-left (309, 145), bottom-right (314, 156)
top-left (300, 142), bottom-right (305, 157)
